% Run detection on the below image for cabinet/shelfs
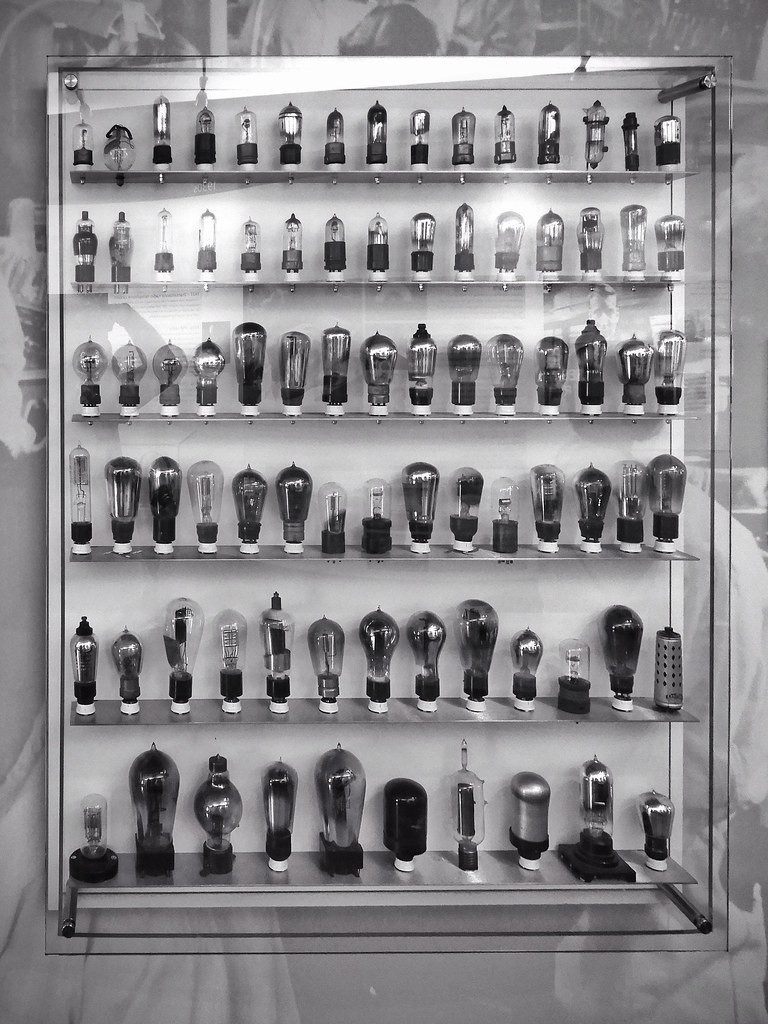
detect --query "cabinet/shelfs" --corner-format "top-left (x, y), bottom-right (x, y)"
top-left (38, 53), bottom-right (732, 957)
